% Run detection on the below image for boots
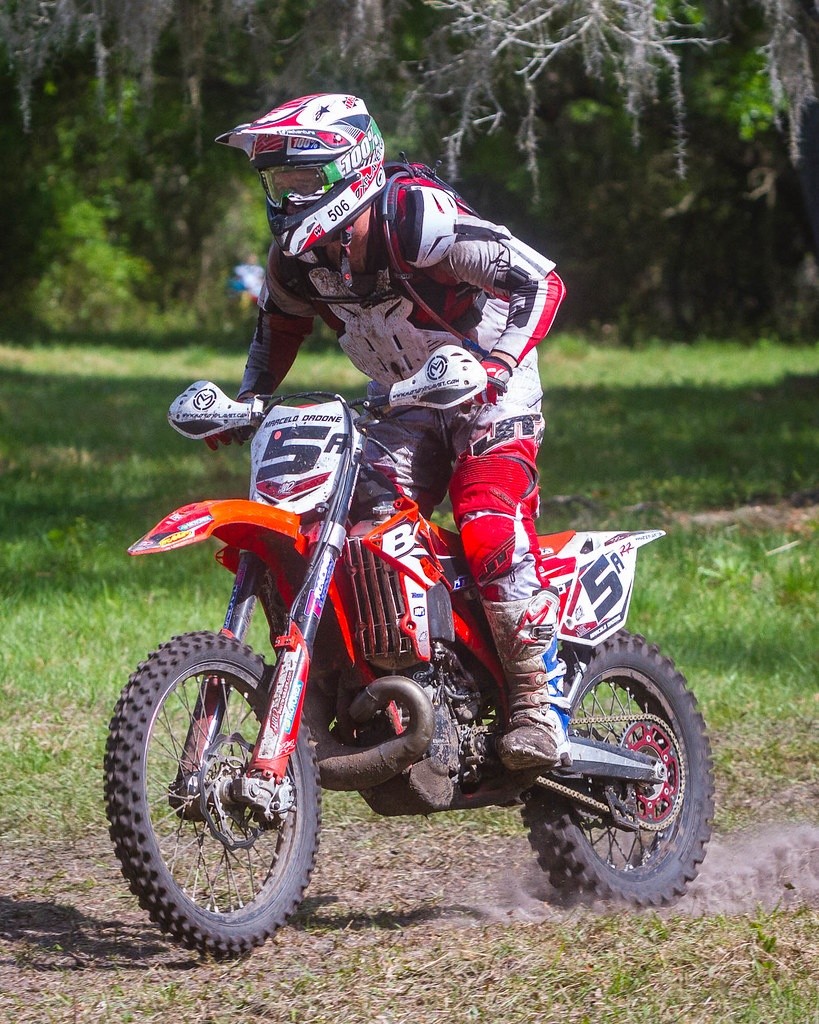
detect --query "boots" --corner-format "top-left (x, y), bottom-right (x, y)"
top-left (480, 585), bottom-right (573, 769)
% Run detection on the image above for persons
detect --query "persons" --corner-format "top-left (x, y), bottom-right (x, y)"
top-left (205, 93), bottom-right (572, 770)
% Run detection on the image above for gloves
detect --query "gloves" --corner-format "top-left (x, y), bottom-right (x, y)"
top-left (459, 356), bottom-right (510, 414)
top-left (205, 397), bottom-right (268, 450)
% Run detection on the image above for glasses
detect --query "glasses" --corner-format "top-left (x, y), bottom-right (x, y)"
top-left (260, 167), bottom-right (334, 208)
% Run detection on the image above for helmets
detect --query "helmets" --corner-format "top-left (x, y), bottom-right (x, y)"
top-left (215, 94), bottom-right (387, 258)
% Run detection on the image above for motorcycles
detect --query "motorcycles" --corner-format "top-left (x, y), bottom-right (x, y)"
top-left (102, 346), bottom-right (716, 958)
